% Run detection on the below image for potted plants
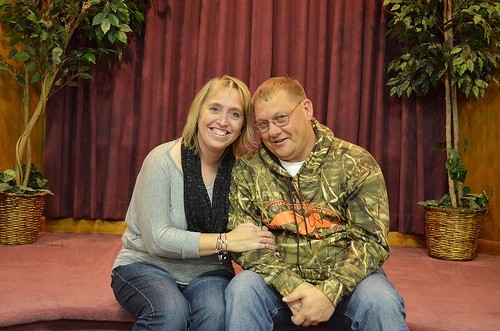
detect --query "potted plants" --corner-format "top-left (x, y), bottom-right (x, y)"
top-left (0, 0), bottom-right (145, 245)
top-left (382, 0), bottom-right (500, 262)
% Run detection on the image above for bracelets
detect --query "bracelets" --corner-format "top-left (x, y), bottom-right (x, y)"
top-left (216, 232), bottom-right (228, 261)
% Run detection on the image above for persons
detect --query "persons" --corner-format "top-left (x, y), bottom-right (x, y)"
top-left (224, 76), bottom-right (409, 331)
top-left (109, 75), bottom-right (276, 331)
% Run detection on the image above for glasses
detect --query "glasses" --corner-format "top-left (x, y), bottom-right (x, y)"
top-left (251, 101), bottom-right (303, 134)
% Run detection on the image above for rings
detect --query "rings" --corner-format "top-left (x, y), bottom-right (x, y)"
top-left (265, 243), bottom-right (267, 248)
